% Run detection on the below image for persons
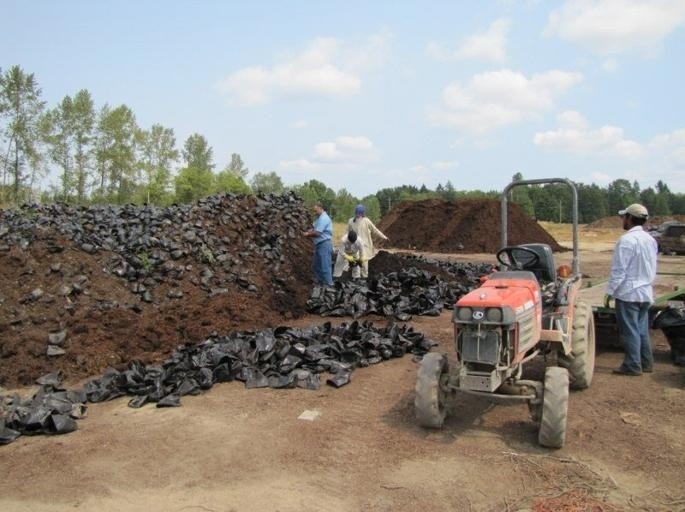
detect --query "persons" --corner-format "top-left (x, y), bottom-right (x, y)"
top-left (331, 229), bottom-right (366, 285)
top-left (602, 202), bottom-right (659, 376)
top-left (345, 204), bottom-right (390, 283)
top-left (300, 201), bottom-right (335, 294)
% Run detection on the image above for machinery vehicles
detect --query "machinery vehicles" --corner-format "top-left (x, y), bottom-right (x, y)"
top-left (407, 179), bottom-right (683, 451)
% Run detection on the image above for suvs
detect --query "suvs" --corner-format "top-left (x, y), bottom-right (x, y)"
top-left (648, 223), bottom-right (685, 256)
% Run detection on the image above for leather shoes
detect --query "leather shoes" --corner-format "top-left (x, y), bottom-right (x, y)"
top-left (612, 368), bottom-right (642, 376)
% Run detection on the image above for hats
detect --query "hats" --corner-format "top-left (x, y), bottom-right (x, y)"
top-left (618, 203), bottom-right (649, 220)
top-left (356, 205), bottom-right (364, 213)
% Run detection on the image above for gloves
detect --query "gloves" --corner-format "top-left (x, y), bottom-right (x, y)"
top-left (360, 256), bottom-right (365, 263)
top-left (604, 294), bottom-right (611, 308)
top-left (346, 256), bottom-right (353, 262)
